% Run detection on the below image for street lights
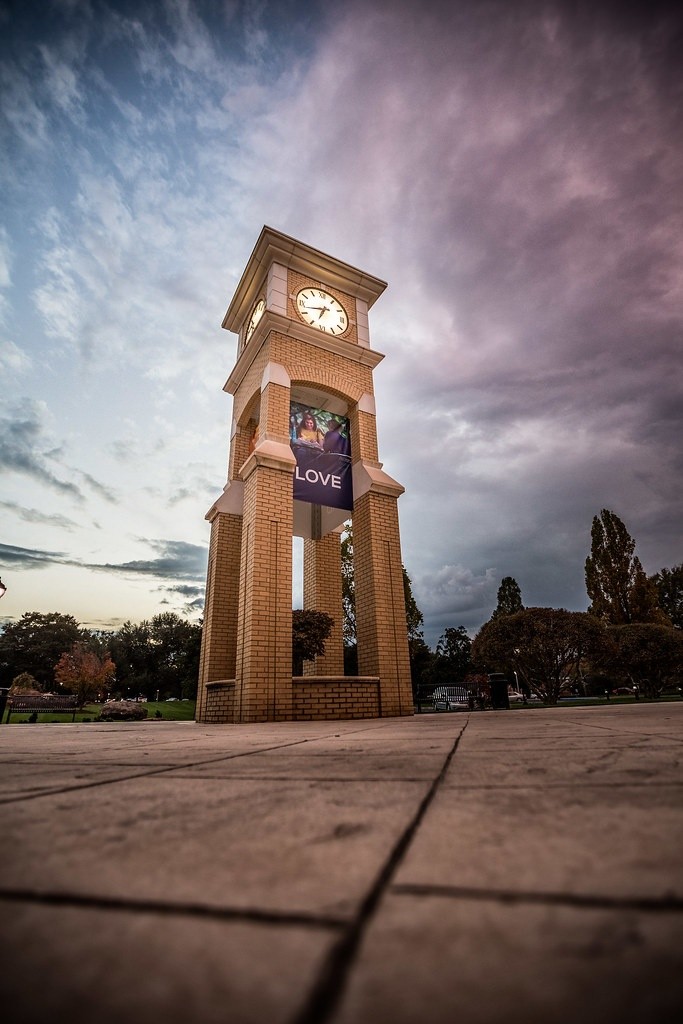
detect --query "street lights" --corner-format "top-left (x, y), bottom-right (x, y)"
top-left (513, 671), bottom-right (519, 692)
top-left (157, 690), bottom-right (159, 702)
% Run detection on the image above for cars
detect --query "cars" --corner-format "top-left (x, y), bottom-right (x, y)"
top-left (613, 687), bottom-right (635, 696)
top-left (166, 698), bottom-right (179, 702)
top-left (508, 691), bottom-right (528, 702)
top-left (467, 690), bottom-right (491, 704)
top-left (530, 690), bottom-right (548, 699)
top-left (433, 686), bottom-right (478, 710)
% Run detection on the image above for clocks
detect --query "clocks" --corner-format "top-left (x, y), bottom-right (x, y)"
top-left (291, 281), bottom-right (357, 339)
top-left (244, 300), bottom-right (265, 346)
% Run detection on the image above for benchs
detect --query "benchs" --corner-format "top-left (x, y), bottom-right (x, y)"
top-left (414, 681), bottom-right (485, 713)
top-left (5, 694), bottom-right (86, 723)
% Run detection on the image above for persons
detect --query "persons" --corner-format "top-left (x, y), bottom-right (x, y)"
top-left (290, 413), bottom-right (349, 456)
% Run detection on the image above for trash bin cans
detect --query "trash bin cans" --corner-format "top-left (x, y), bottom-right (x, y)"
top-left (487, 673), bottom-right (510, 710)
